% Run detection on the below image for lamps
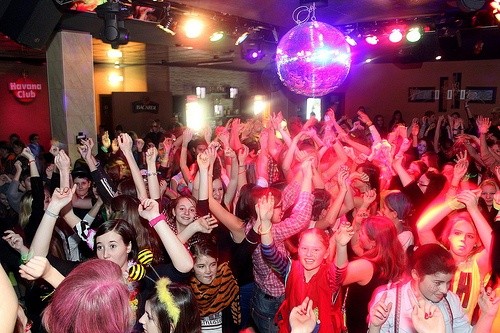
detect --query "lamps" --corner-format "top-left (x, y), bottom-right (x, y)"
top-left (156, 2), bottom-right (178, 35)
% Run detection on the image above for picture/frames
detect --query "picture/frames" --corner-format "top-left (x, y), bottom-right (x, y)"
top-left (464, 86), bottom-right (497, 104)
top-left (407, 86), bottom-right (435, 102)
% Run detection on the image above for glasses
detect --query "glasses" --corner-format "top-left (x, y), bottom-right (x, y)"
top-left (274, 202), bottom-right (284, 211)
top-left (417, 183), bottom-right (429, 187)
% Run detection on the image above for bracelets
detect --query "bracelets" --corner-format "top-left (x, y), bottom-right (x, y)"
top-left (208, 173), bottom-right (214, 177)
top-left (258, 223), bottom-right (272, 235)
top-left (45, 210), bottom-right (59, 219)
top-left (85, 159), bottom-right (93, 163)
top-left (446, 193), bottom-right (457, 199)
top-left (333, 119), bottom-right (335, 123)
top-left (147, 173), bottom-right (158, 177)
top-left (161, 159), bottom-right (170, 162)
top-left (237, 165), bottom-right (247, 167)
top-left (238, 169), bottom-right (247, 176)
top-left (101, 146), bottom-right (108, 153)
top-left (366, 121), bottom-right (372, 125)
top-left (244, 234), bottom-right (258, 246)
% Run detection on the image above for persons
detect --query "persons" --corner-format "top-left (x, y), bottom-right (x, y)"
top-left (0, 105), bottom-right (500, 333)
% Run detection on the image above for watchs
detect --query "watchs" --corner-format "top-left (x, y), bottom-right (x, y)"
top-left (454, 129), bottom-right (459, 130)
top-left (449, 185), bottom-right (459, 191)
top-left (27, 160), bottom-right (37, 166)
top-left (186, 180), bottom-right (193, 183)
top-left (148, 213), bottom-right (167, 228)
top-left (460, 129), bottom-right (466, 131)
top-left (95, 162), bottom-right (100, 167)
